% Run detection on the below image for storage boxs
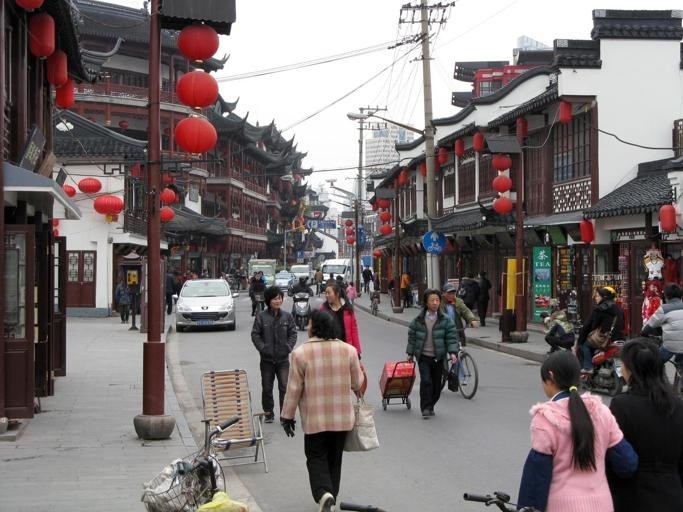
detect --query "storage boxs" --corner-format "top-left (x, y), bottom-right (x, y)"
top-left (379, 360), bottom-right (416, 398)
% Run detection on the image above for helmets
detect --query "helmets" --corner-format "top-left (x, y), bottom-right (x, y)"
top-left (597, 286), bottom-right (617, 302)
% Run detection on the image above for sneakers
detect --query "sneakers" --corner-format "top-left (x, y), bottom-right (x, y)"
top-left (580, 368), bottom-right (594, 374)
top-left (319, 491), bottom-right (336, 512)
top-left (263, 412), bottom-right (276, 424)
top-left (420, 409), bottom-right (436, 417)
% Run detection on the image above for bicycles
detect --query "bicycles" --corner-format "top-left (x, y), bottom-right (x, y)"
top-left (141, 413), bottom-right (243, 510)
top-left (637, 331), bottom-right (683, 405)
top-left (444, 323), bottom-right (482, 400)
top-left (327, 488), bottom-right (520, 512)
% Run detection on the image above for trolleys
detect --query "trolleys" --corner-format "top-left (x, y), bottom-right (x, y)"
top-left (380, 361), bottom-right (416, 414)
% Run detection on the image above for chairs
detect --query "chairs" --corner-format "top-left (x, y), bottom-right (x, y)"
top-left (200, 369), bottom-right (268, 477)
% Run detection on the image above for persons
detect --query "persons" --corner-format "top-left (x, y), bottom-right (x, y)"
top-left (226, 264), bottom-right (413, 423)
top-left (438, 283), bottom-right (479, 379)
top-left (165, 267), bottom-right (209, 314)
top-left (475, 270), bottom-right (492, 326)
top-left (405, 289), bottom-right (459, 416)
top-left (517, 281), bottom-right (682, 509)
top-left (115, 276), bottom-right (131, 324)
top-left (458, 273), bottom-right (480, 327)
top-left (280, 309), bottom-right (364, 512)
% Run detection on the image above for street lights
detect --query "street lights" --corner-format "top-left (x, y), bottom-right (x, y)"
top-left (318, 197), bottom-right (363, 297)
top-left (283, 223), bottom-right (309, 272)
top-left (482, 130), bottom-right (529, 337)
top-left (139, 1), bottom-right (236, 412)
top-left (343, 109), bottom-right (440, 310)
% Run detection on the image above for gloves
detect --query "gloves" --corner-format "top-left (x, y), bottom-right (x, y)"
top-left (280, 418), bottom-right (296, 438)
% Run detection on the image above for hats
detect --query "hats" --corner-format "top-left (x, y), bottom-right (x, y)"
top-left (442, 283), bottom-right (456, 294)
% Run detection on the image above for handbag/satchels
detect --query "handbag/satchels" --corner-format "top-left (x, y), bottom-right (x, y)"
top-left (447, 373), bottom-right (459, 392)
top-left (586, 327), bottom-right (610, 350)
top-left (343, 391), bottom-right (380, 452)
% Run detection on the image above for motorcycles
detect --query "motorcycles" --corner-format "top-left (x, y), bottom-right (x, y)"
top-left (286, 286), bottom-right (314, 335)
top-left (568, 317), bottom-right (625, 393)
top-left (369, 288), bottom-right (381, 316)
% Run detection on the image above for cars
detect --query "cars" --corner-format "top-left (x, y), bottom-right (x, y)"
top-left (275, 263), bottom-right (319, 293)
top-left (171, 276), bottom-right (240, 334)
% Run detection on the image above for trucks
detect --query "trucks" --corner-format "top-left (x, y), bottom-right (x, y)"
top-left (320, 258), bottom-right (367, 293)
top-left (245, 257), bottom-right (277, 293)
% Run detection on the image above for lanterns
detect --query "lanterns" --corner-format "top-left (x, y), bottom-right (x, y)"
top-left (490, 156), bottom-right (513, 214)
top-left (345, 219), bottom-right (354, 245)
top-left (516, 117), bottom-right (528, 139)
top-left (17, 0), bottom-right (74, 110)
top-left (373, 250), bottom-right (382, 257)
top-left (375, 192), bottom-right (391, 236)
top-left (52, 161), bottom-right (177, 240)
top-left (399, 132), bottom-right (483, 184)
top-left (119, 120), bottom-right (128, 128)
top-left (660, 204), bottom-right (676, 232)
top-left (559, 100), bottom-right (571, 123)
top-left (174, 22), bottom-right (219, 152)
top-left (580, 219), bottom-right (594, 244)
